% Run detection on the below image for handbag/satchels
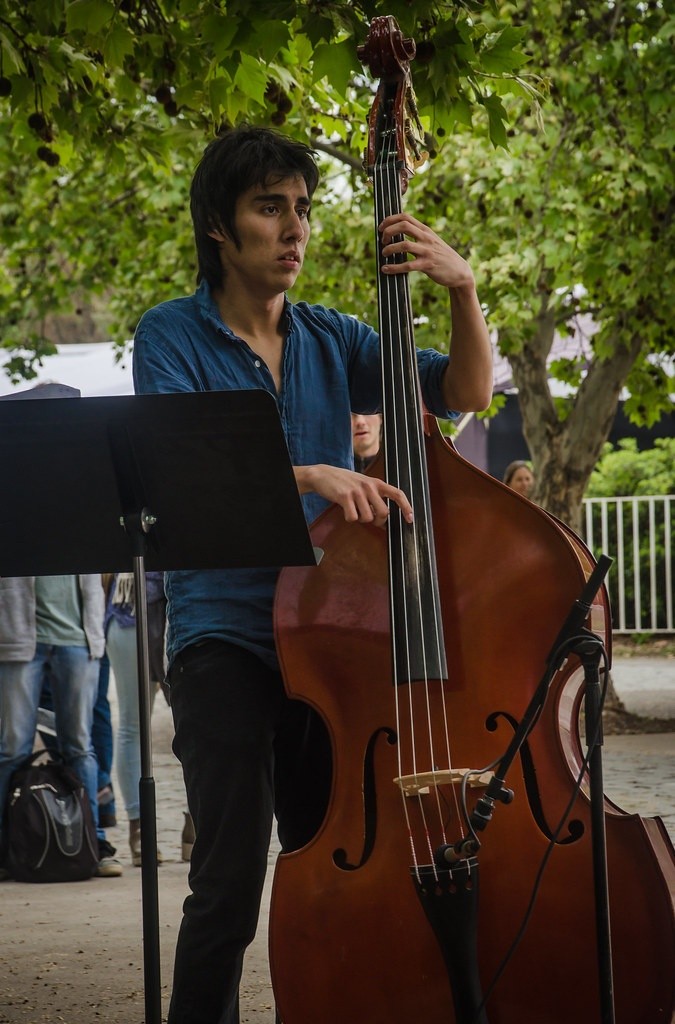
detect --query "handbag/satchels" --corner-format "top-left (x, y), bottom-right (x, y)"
top-left (0, 750), bottom-right (98, 884)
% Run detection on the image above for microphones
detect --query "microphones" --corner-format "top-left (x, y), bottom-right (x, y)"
top-left (433, 837), bottom-right (481, 870)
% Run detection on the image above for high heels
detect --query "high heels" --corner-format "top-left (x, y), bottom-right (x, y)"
top-left (182, 811), bottom-right (198, 860)
top-left (130, 820), bottom-right (162, 866)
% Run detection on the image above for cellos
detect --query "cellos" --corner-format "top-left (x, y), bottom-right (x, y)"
top-left (269, 16), bottom-right (673, 1024)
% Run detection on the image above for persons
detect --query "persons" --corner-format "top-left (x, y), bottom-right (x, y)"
top-left (504, 460), bottom-right (536, 500)
top-left (0, 572), bottom-right (195, 878)
top-left (133, 124), bottom-right (494, 1024)
top-left (350, 411), bottom-right (383, 474)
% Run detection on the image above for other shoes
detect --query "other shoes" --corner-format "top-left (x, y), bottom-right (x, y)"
top-left (97, 837), bottom-right (123, 875)
top-left (98, 815), bottom-right (117, 828)
